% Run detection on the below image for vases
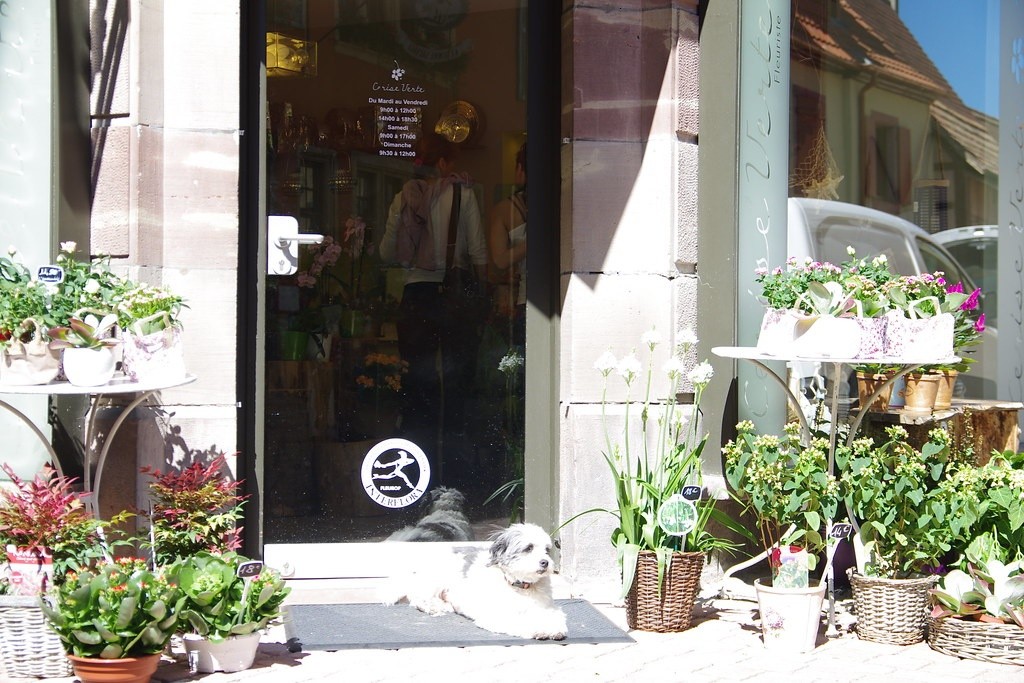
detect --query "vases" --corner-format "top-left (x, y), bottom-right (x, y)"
top-left (346, 402), bottom-right (397, 440)
top-left (845, 572), bottom-right (946, 644)
top-left (627, 551), bottom-right (705, 632)
top-left (926, 368), bottom-right (960, 409)
top-left (338, 302), bottom-right (371, 337)
top-left (854, 372), bottom-right (894, 411)
top-left (905, 372), bottom-right (941, 411)
top-left (929, 612), bottom-right (1023, 665)
top-left (178, 631), bottom-right (266, 673)
top-left (755, 580), bottom-right (822, 650)
top-left (68, 650), bottom-right (165, 682)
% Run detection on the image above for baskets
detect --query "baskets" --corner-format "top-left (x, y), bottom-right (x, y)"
top-left (844, 565), bottom-right (941, 646)
top-left (619, 548), bottom-right (704, 632)
top-left (924, 601), bottom-right (1024, 667)
top-left (1, 594), bottom-right (74, 679)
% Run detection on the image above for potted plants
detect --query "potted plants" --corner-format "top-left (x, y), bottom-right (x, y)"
top-left (47, 311), bottom-right (118, 386)
top-left (275, 307), bottom-right (329, 359)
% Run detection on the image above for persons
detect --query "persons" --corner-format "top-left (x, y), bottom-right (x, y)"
top-left (380, 134), bottom-right (490, 438)
top-left (485, 143), bottom-right (526, 441)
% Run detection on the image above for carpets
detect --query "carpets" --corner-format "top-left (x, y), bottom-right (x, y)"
top-left (284, 598), bottom-right (636, 652)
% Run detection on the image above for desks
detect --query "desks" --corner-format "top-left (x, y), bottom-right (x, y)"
top-left (0, 372), bottom-right (199, 556)
top-left (709, 346), bottom-right (964, 633)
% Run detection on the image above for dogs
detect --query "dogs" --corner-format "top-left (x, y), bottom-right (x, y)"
top-left (382, 522), bottom-right (569, 640)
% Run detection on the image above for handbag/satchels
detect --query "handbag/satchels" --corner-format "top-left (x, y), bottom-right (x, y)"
top-left (436, 266), bottom-right (489, 321)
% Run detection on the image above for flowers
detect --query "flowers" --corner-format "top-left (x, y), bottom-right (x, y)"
top-left (908, 441), bottom-right (1024, 622)
top-left (843, 420), bottom-right (966, 578)
top-left (350, 354), bottom-right (414, 405)
top-left (0, 245), bottom-right (178, 340)
top-left (547, 332), bottom-right (759, 602)
top-left (164, 552), bottom-right (290, 637)
top-left (764, 251), bottom-right (986, 369)
top-left (295, 215), bottom-right (383, 300)
top-left (718, 417), bottom-right (853, 588)
top-left (45, 553), bottom-right (187, 656)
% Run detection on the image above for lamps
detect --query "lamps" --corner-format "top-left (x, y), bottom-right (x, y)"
top-left (265, 32), bottom-right (321, 79)
